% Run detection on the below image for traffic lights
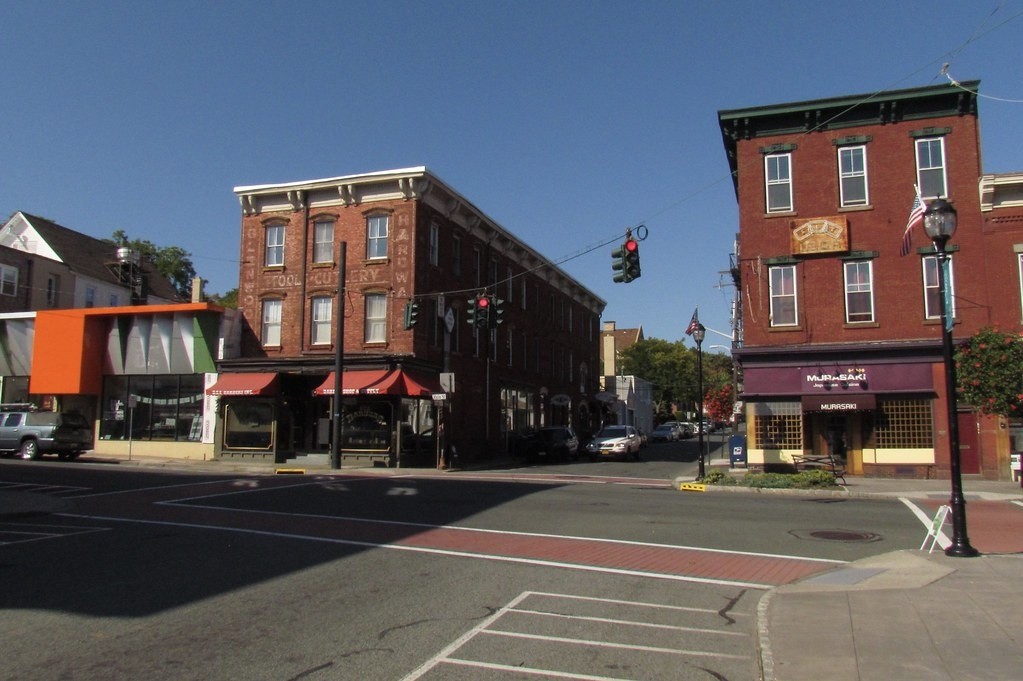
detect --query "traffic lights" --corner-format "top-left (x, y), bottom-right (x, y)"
top-left (624, 238), bottom-right (641, 279)
top-left (467, 297), bottom-right (477, 328)
top-left (611, 243), bottom-right (629, 284)
top-left (477, 297), bottom-right (490, 329)
top-left (492, 297), bottom-right (505, 328)
top-left (407, 301), bottom-right (421, 328)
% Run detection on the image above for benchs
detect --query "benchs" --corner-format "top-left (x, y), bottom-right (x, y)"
top-left (791, 454), bottom-right (847, 485)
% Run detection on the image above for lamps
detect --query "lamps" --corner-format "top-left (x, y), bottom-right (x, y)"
top-left (851, 362), bottom-right (859, 376)
top-left (815, 360), bottom-right (823, 377)
top-left (832, 360), bottom-right (839, 377)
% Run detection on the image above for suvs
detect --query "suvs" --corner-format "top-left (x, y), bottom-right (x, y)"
top-left (0, 402), bottom-right (95, 462)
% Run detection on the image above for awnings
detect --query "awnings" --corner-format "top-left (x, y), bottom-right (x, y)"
top-left (313, 369), bottom-right (445, 396)
top-left (206, 372), bottom-right (280, 395)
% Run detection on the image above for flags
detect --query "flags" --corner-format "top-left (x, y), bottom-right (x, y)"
top-left (685, 308), bottom-right (698, 336)
top-left (900, 194), bottom-right (923, 257)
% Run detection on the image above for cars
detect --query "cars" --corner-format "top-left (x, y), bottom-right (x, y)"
top-left (652, 417), bottom-right (732, 444)
top-left (585, 424), bottom-right (649, 462)
top-left (534, 423), bottom-right (580, 464)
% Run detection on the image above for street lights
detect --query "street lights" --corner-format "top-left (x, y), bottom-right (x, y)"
top-left (922, 192), bottom-right (979, 558)
top-left (692, 319), bottom-right (707, 483)
